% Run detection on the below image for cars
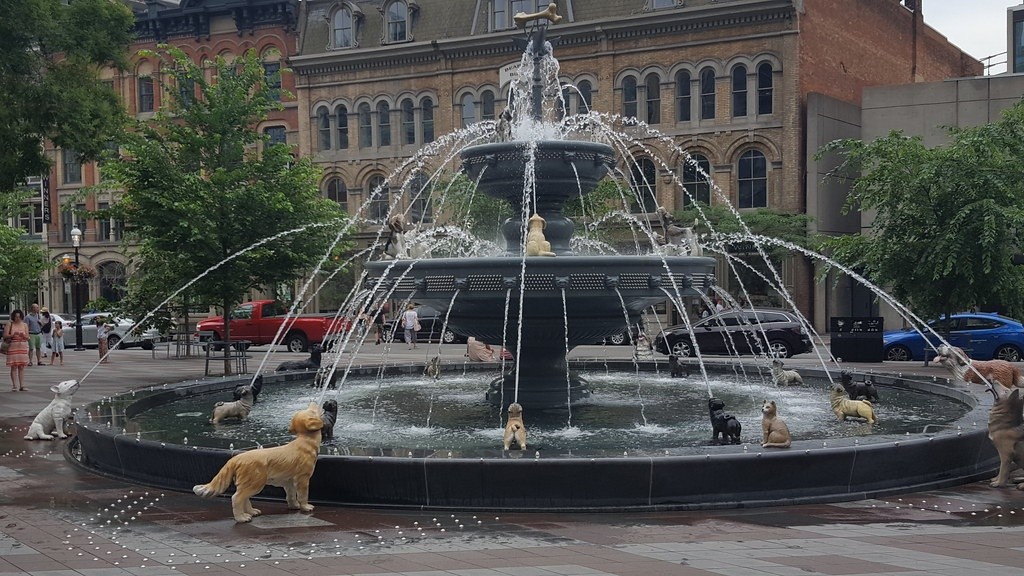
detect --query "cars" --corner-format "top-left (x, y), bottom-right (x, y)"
top-left (881, 310), bottom-right (1024, 362)
top-left (0, 313), bottom-right (156, 350)
top-left (596, 317), bottom-right (644, 346)
top-left (382, 305), bottom-right (468, 346)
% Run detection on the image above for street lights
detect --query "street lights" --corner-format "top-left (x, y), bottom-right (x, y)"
top-left (70, 224), bottom-right (84, 351)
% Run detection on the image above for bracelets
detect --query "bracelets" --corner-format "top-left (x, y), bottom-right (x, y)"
top-left (27, 337), bottom-right (30, 340)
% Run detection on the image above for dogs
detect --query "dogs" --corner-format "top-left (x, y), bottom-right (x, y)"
top-left (491, 106), bottom-right (512, 143)
top-left (708, 396), bottom-right (742, 440)
top-left (589, 114), bottom-right (610, 144)
top-left (380, 212), bottom-right (432, 260)
top-left (504, 402), bottom-right (526, 451)
top-left (428, 357), bottom-right (442, 380)
top-left (838, 370), bottom-right (880, 401)
top-left (650, 207), bottom-right (703, 256)
top-left (934, 344), bottom-right (1024, 389)
top-left (526, 213), bottom-right (556, 257)
top-left (760, 399), bottom-right (791, 447)
top-left (984, 379), bottom-right (1024, 490)
top-left (24, 380), bottom-right (80, 440)
top-left (668, 355), bottom-right (690, 378)
top-left (636, 332), bottom-right (654, 359)
top-left (234, 374), bottom-right (264, 402)
top-left (829, 383), bottom-right (877, 424)
top-left (314, 364), bottom-right (337, 390)
top-left (276, 344), bottom-right (327, 372)
top-left (192, 385), bottom-right (338, 523)
top-left (773, 359), bottom-right (804, 387)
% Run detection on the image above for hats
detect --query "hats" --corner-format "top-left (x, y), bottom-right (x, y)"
top-left (40, 307), bottom-right (48, 312)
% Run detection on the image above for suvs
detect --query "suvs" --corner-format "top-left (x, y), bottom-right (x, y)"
top-left (656, 308), bottom-right (816, 358)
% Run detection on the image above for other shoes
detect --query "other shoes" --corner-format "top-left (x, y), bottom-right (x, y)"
top-left (12, 387), bottom-right (17, 392)
top-left (19, 387), bottom-right (28, 391)
top-left (27, 352), bottom-right (64, 366)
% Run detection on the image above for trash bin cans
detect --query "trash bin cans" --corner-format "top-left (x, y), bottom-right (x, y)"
top-left (830, 317), bottom-right (883, 363)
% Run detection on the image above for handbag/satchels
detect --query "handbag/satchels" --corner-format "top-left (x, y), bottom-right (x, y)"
top-left (413, 318), bottom-right (421, 331)
top-left (0, 322), bottom-right (13, 354)
top-left (401, 313), bottom-right (407, 328)
top-left (41, 317), bottom-right (51, 335)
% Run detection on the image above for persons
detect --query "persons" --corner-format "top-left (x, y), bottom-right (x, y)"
top-left (702, 309), bottom-right (709, 318)
top-left (3, 309), bottom-right (30, 391)
top-left (716, 301), bottom-right (723, 313)
top-left (47, 321), bottom-right (64, 365)
top-left (96, 315), bottom-right (110, 363)
top-left (402, 304), bottom-right (417, 350)
top-left (370, 303), bottom-right (386, 345)
top-left (24, 304), bottom-right (46, 366)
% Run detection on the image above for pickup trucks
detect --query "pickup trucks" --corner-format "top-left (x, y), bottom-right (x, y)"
top-left (194, 301), bottom-right (353, 353)
